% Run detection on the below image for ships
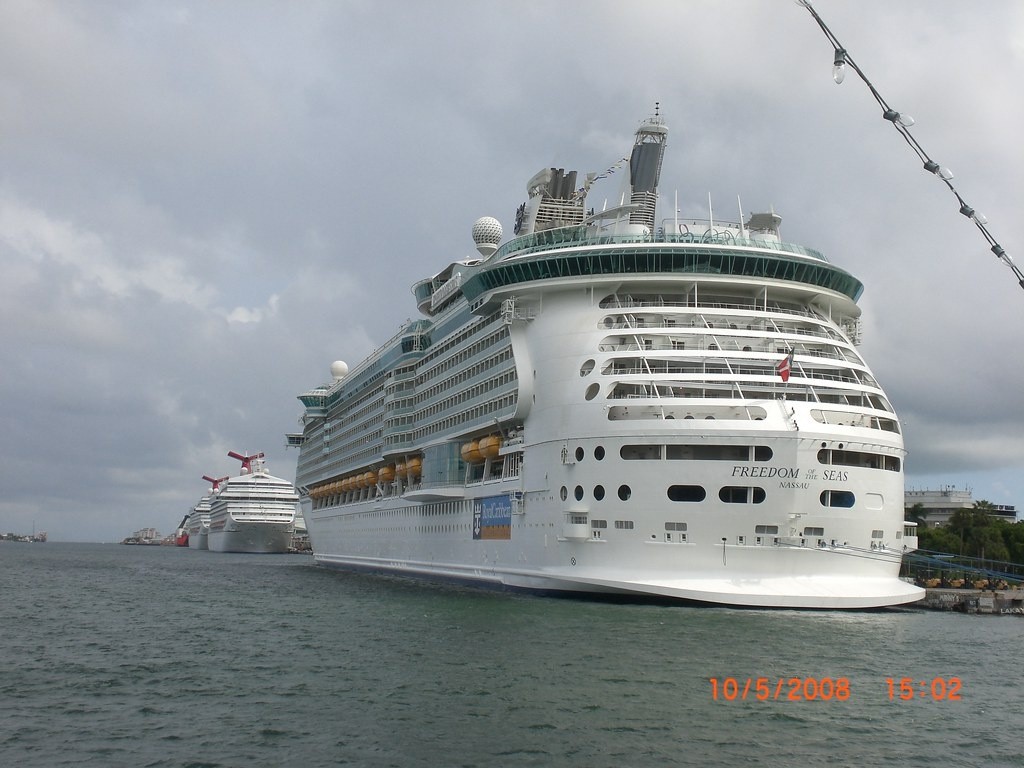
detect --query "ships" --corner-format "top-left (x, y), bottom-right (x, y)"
top-left (201, 450), bottom-right (299, 554)
top-left (283, 115), bottom-right (930, 608)
top-left (189, 497), bottom-right (211, 550)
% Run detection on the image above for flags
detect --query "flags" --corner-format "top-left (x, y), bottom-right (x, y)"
top-left (777, 347), bottom-right (794, 382)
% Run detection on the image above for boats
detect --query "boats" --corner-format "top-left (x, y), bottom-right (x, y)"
top-left (364, 471), bottom-right (377, 486)
top-left (174, 514), bottom-right (191, 547)
top-left (405, 457), bottom-right (420, 475)
top-left (478, 436), bottom-right (502, 457)
top-left (323, 484), bottom-right (330, 493)
top-left (356, 474), bottom-right (365, 489)
top-left (396, 462), bottom-right (406, 477)
top-left (330, 482), bottom-right (336, 492)
top-left (349, 476), bottom-right (356, 488)
top-left (460, 439), bottom-right (479, 461)
top-left (335, 481), bottom-right (342, 491)
top-left (319, 485), bottom-right (324, 496)
top-left (342, 479), bottom-right (349, 491)
top-left (309, 489), bottom-right (314, 497)
top-left (314, 488), bottom-right (319, 497)
top-left (378, 467), bottom-right (395, 481)
